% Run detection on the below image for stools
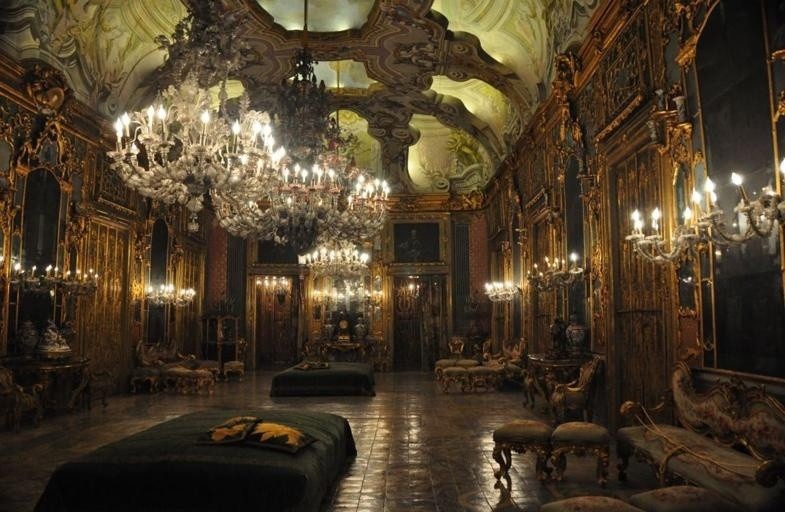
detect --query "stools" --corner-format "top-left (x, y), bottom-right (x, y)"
top-left (632, 479), bottom-right (748, 512)
top-left (494, 417), bottom-right (555, 484)
top-left (432, 333), bottom-right (608, 422)
top-left (123, 359), bottom-right (248, 397)
top-left (535, 478), bottom-right (643, 511)
top-left (549, 420), bottom-right (611, 488)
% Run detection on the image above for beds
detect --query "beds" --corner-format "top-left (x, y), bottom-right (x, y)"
top-left (267, 361), bottom-right (376, 398)
top-left (33, 406), bottom-right (356, 505)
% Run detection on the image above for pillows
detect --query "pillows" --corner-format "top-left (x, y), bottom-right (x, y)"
top-left (244, 420), bottom-right (322, 455)
top-left (313, 364), bottom-right (329, 369)
top-left (294, 364), bottom-right (313, 371)
top-left (194, 411), bottom-right (263, 449)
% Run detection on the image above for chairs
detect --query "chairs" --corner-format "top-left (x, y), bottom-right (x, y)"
top-left (0, 366), bottom-right (45, 432)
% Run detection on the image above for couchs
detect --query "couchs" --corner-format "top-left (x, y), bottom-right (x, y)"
top-left (617, 359), bottom-right (784, 512)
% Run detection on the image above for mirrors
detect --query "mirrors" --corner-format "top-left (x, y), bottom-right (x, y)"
top-left (216, 312), bottom-right (239, 364)
top-left (17, 162), bottom-right (62, 357)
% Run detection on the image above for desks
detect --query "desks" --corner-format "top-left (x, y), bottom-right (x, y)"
top-left (17, 355), bottom-right (91, 416)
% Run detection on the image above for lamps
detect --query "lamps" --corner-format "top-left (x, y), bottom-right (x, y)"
top-left (43, 250), bottom-right (99, 299)
top-left (297, 243), bottom-right (384, 307)
top-left (254, 275), bottom-right (288, 301)
top-left (406, 269), bottom-right (422, 299)
top-left (105, 71), bottom-right (389, 243)
top-left (525, 253), bottom-right (588, 292)
top-left (20, 261), bottom-right (41, 297)
top-left (624, 158), bottom-right (785, 272)
top-left (145, 284), bottom-right (196, 308)
top-left (484, 278), bottom-right (519, 303)
